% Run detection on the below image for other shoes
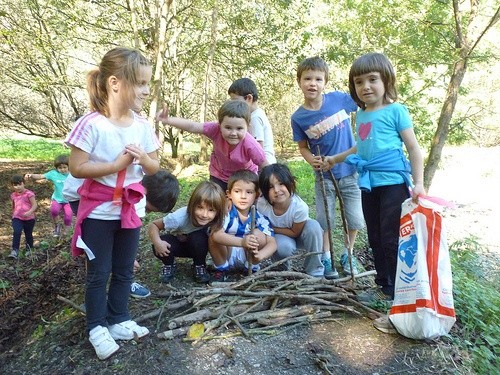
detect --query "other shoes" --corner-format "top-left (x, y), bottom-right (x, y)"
top-left (244, 266), bottom-right (259, 276)
top-left (214, 269), bottom-right (229, 279)
top-left (133, 261), bottom-right (141, 272)
top-left (371, 289), bottom-right (393, 301)
top-left (52, 225), bottom-right (62, 238)
top-left (8, 250), bottom-right (18, 259)
top-left (25, 251), bottom-right (31, 257)
top-left (372, 315), bottom-right (398, 334)
top-left (64, 226), bottom-right (70, 237)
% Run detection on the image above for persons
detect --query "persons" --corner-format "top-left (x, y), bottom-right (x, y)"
top-left (64, 46), bottom-right (162, 361)
top-left (228, 78), bottom-right (276, 169)
top-left (206, 169), bottom-right (278, 287)
top-left (319, 51), bottom-right (427, 334)
top-left (159, 101), bottom-right (269, 196)
top-left (148, 181), bottom-right (226, 284)
top-left (63, 170), bottom-right (180, 299)
top-left (23, 156), bottom-right (73, 239)
top-left (290, 56), bottom-right (366, 280)
top-left (7, 175), bottom-right (38, 260)
top-left (225, 163), bottom-right (325, 278)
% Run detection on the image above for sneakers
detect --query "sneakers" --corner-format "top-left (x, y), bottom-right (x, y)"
top-left (192, 263), bottom-right (211, 284)
top-left (320, 258), bottom-right (338, 278)
top-left (130, 282), bottom-right (151, 298)
top-left (107, 320), bottom-right (150, 341)
top-left (88, 325), bottom-right (120, 361)
top-left (158, 260), bottom-right (178, 283)
top-left (340, 254), bottom-right (358, 277)
